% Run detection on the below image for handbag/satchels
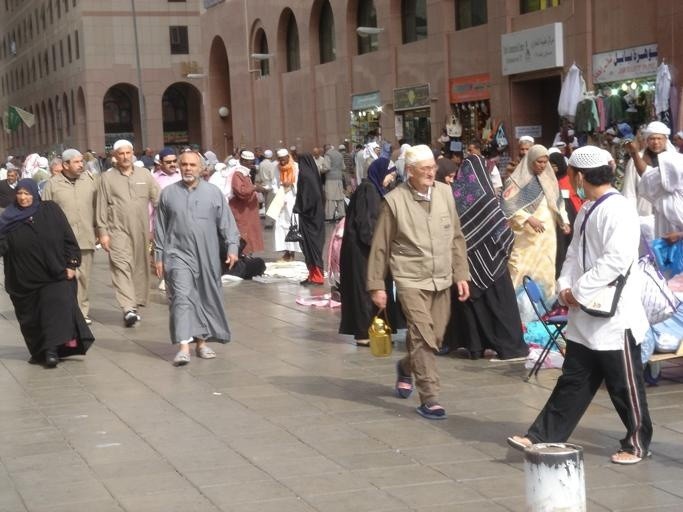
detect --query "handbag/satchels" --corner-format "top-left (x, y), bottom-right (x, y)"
top-left (638, 249), bottom-right (682, 325)
top-left (579, 284), bottom-right (622, 318)
top-left (368, 323), bottom-right (392, 356)
top-left (285, 213), bottom-right (305, 243)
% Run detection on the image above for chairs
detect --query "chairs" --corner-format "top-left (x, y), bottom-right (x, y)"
top-left (523, 275), bottom-right (567, 380)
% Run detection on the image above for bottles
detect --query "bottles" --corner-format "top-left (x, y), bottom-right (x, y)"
top-left (367, 317), bottom-right (392, 358)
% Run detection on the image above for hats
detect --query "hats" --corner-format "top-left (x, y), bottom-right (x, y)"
top-left (60, 149), bottom-right (84, 163)
top-left (158, 148), bottom-right (176, 158)
top-left (518, 135), bottom-right (534, 145)
top-left (240, 148), bottom-right (288, 160)
top-left (404, 144), bottom-right (434, 167)
top-left (567, 146), bottom-right (616, 169)
top-left (112, 139), bottom-right (133, 154)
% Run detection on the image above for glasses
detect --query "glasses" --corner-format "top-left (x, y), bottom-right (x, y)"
top-left (162, 159), bottom-right (177, 164)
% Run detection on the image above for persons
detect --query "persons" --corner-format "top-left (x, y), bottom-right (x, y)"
top-left (361, 143), bottom-right (471, 421)
top-left (1, 178), bottom-right (93, 368)
top-left (503, 144), bottom-right (655, 466)
top-left (1, 121), bottom-right (681, 329)
top-left (339, 156), bottom-right (402, 348)
top-left (154, 145), bottom-right (241, 367)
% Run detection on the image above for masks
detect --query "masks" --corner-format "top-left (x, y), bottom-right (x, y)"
top-left (576, 176), bottom-right (588, 201)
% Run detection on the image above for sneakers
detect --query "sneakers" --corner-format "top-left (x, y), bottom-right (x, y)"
top-left (122, 312), bottom-right (137, 327)
top-left (195, 346), bottom-right (216, 359)
top-left (173, 344), bottom-right (190, 364)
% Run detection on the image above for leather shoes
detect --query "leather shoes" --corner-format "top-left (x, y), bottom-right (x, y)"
top-left (45, 351), bottom-right (56, 366)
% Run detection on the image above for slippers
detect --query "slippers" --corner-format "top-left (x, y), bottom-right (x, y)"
top-left (610, 449), bottom-right (642, 464)
top-left (507, 435), bottom-right (532, 449)
top-left (416, 401), bottom-right (447, 419)
top-left (397, 358), bottom-right (413, 398)
